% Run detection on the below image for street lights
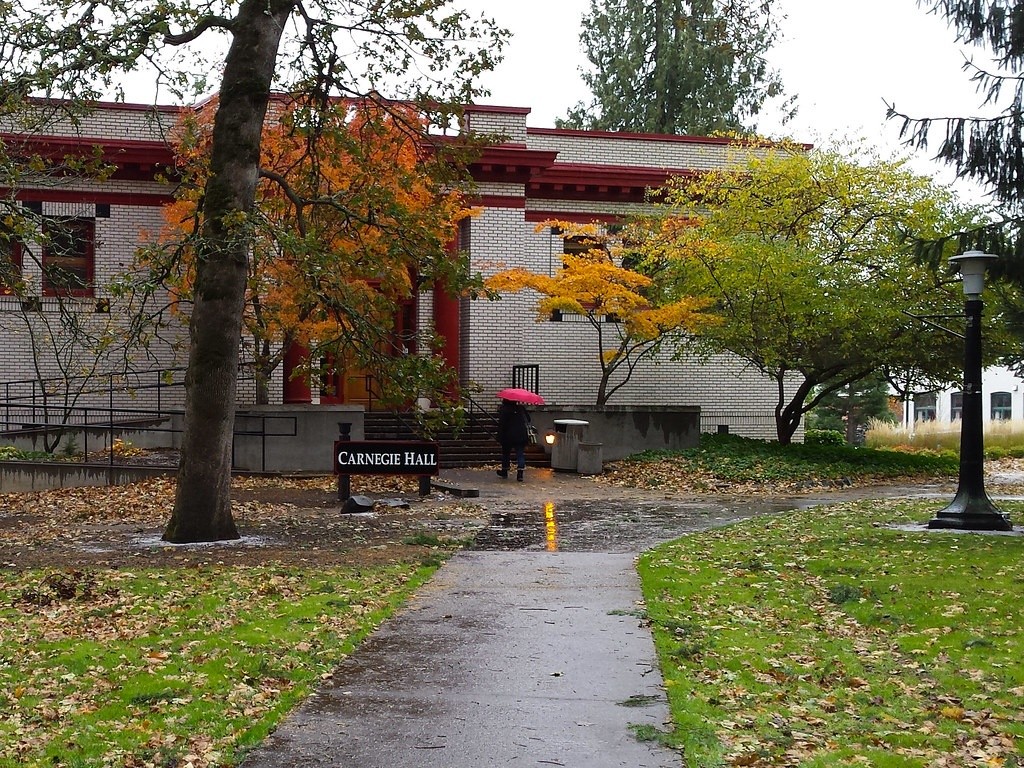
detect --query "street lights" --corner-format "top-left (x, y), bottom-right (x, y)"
top-left (926, 250), bottom-right (1016, 531)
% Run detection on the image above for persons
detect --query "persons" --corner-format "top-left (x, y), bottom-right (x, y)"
top-left (497, 398), bottom-right (525, 482)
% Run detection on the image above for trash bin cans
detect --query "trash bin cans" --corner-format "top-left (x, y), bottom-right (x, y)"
top-left (577, 441), bottom-right (603, 475)
top-left (551, 419), bottom-right (590, 473)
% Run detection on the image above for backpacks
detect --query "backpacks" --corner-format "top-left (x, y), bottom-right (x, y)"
top-left (524, 414), bottom-right (538, 444)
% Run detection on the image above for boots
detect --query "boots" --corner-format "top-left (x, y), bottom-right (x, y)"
top-left (497, 466), bottom-right (511, 478)
top-left (517, 464), bottom-right (527, 481)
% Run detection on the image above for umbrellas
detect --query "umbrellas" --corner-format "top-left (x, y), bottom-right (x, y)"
top-left (495, 388), bottom-right (545, 405)
